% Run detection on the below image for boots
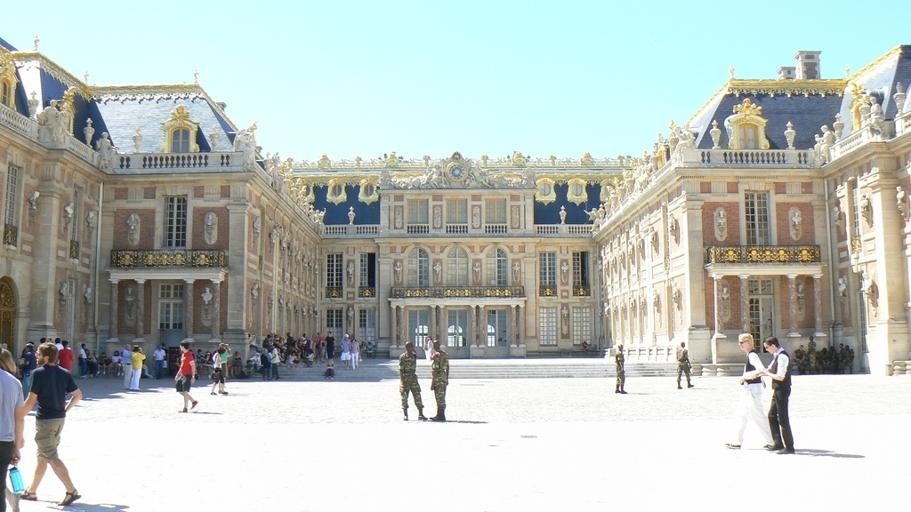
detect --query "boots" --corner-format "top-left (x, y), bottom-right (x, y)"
top-left (677, 380), bottom-right (694, 390)
top-left (402, 407), bottom-right (449, 421)
top-left (615, 385), bottom-right (628, 393)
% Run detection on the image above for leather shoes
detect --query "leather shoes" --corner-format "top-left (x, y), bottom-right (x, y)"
top-left (726, 441), bottom-right (794, 455)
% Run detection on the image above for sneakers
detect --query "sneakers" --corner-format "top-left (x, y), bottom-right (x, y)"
top-left (176, 391), bottom-right (229, 412)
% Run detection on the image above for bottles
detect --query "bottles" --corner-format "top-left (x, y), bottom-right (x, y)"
top-left (10, 466), bottom-right (25, 494)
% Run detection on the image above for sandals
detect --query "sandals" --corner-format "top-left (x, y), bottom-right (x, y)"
top-left (20, 489), bottom-right (40, 501)
top-left (58, 488), bottom-right (83, 507)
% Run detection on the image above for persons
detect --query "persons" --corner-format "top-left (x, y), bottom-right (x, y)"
top-left (614, 343), bottom-right (629, 394)
top-left (725, 333), bottom-right (776, 450)
top-left (814, 125), bottom-right (832, 152)
top-left (584, 119), bottom-right (697, 230)
top-left (430, 340), bottom-right (450, 422)
top-left (792, 335), bottom-right (855, 373)
top-left (863, 97), bottom-right (882, 129)
top-left (398, 341), bottom-right (428, 420)
top-left (0, 347), bottom-right (26, 512)
top-left (22, 341), bottom-right (83, 506)
top-left (174, 340), bottom-right (200, 414)
top-left (753, 336), bottom-right (797, 454)
top-left (234, 123), bottom-right (327, 225)
top-left (676, 342), bottom-right (695, 389)
